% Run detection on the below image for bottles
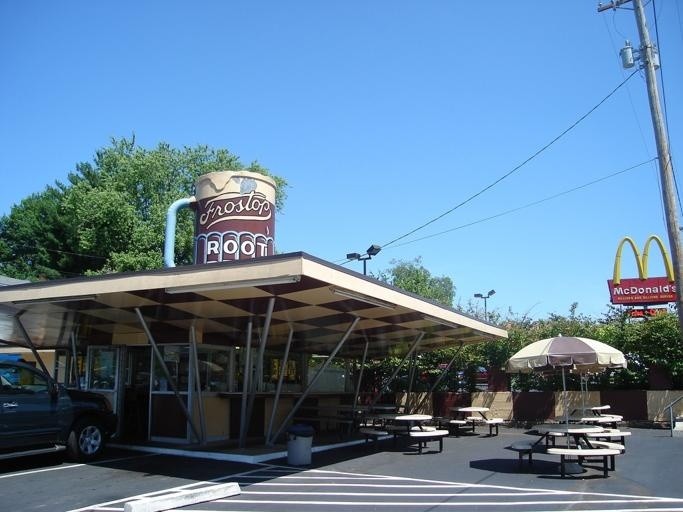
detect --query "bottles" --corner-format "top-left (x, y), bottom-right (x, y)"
top-left (282, 380), bottom-right (300, 392)
top-left (210, 379), bottom-right (216, 392)
top-left (263, 375), bottom-right (270, 391)
top-left (158, 376), bottom-right (167, 391)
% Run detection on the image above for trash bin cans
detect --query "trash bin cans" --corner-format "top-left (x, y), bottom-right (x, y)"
top-left (284, 424), bottom-right (317, 466)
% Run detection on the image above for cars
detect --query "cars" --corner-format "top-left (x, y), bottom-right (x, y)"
top-left (0, 353), bottom-right (118, 474)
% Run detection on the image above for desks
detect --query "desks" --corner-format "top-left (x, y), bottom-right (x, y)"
top-left (571, 405), bottom-right (611, 415)
top-left (378, 413), bottom-right (433, 431)
top-left (449, 406), bottom-right (489, 420)
top-left (341, 405), bottom-right (396, 414)
top-left (365, 416), bottom-right (378, 426)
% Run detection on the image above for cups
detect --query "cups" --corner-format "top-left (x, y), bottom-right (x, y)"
top-left (163, 169), bottom-right (277, 266)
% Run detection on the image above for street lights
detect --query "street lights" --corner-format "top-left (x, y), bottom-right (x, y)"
top-left (472, 290), bottom-right (496, 322)
top-left (346, 244), bottom-right (381, 276)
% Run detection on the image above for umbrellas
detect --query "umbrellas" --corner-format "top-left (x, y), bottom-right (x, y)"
top-left (504, 333), bottom-right (628, 449)
top-left (543, 359), bottom-right (627, 416)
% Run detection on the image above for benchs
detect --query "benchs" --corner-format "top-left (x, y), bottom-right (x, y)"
top-left (402, 429), bottom-right (449, 455)
top-left (503, 414), bottom-right (632, 479)
top-left (360, 423), bottom-right (381, 427)
top-left (388, 426), bottom-right (436, 447)
top-left (445, 418), bottom-right (467, 436)
top-left (467, 417), bottom-right (503, 437)
top-left (360, 427), bottom-right (388, 445)
